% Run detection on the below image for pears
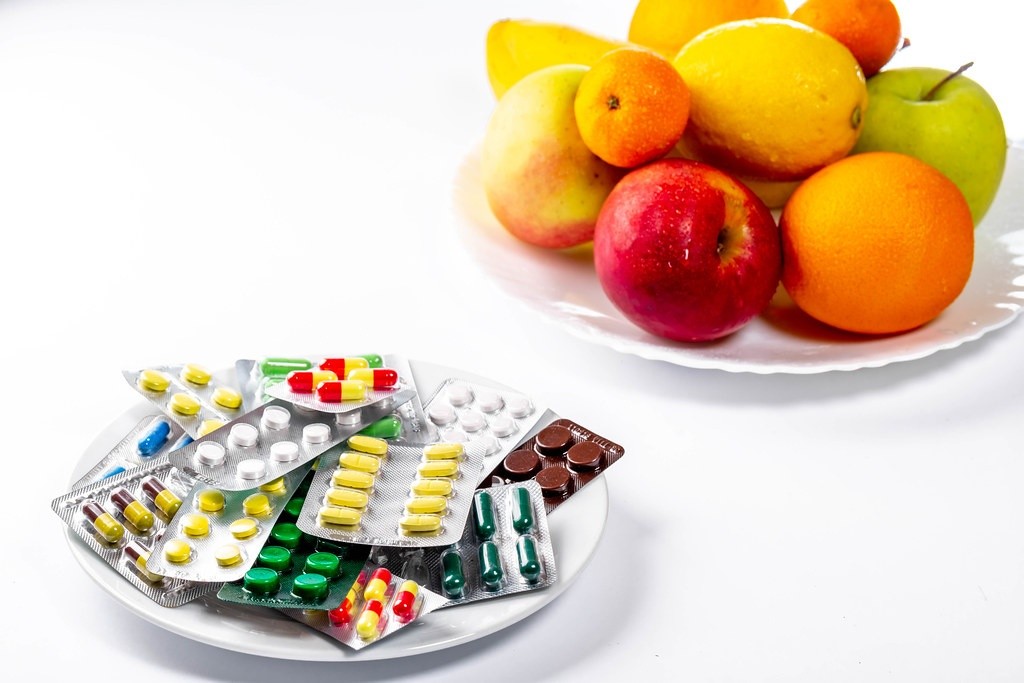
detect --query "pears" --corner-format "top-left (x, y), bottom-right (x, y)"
top-left (849, 61), bottom-right (1008, 231)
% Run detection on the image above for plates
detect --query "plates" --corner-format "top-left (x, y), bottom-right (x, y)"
top-left (61, 373), bottom-right (609, 662)
top-left (443, 131), bottom-right (1024, 375)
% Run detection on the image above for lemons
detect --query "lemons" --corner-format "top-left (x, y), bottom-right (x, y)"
top-left (673, 18), bottom-right (868, 182)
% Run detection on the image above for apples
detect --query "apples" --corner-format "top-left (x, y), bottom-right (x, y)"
top-left (480, 62), bottom-right (782, 342)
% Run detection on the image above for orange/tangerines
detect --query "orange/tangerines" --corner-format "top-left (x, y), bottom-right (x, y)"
top-left (574, 45), bottom-right (691, 170)
top-left (782, 150), bottom-right (977, 336)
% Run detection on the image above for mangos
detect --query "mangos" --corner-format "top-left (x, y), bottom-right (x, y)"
top-left (484, 0), bottom-right (900, 98)
